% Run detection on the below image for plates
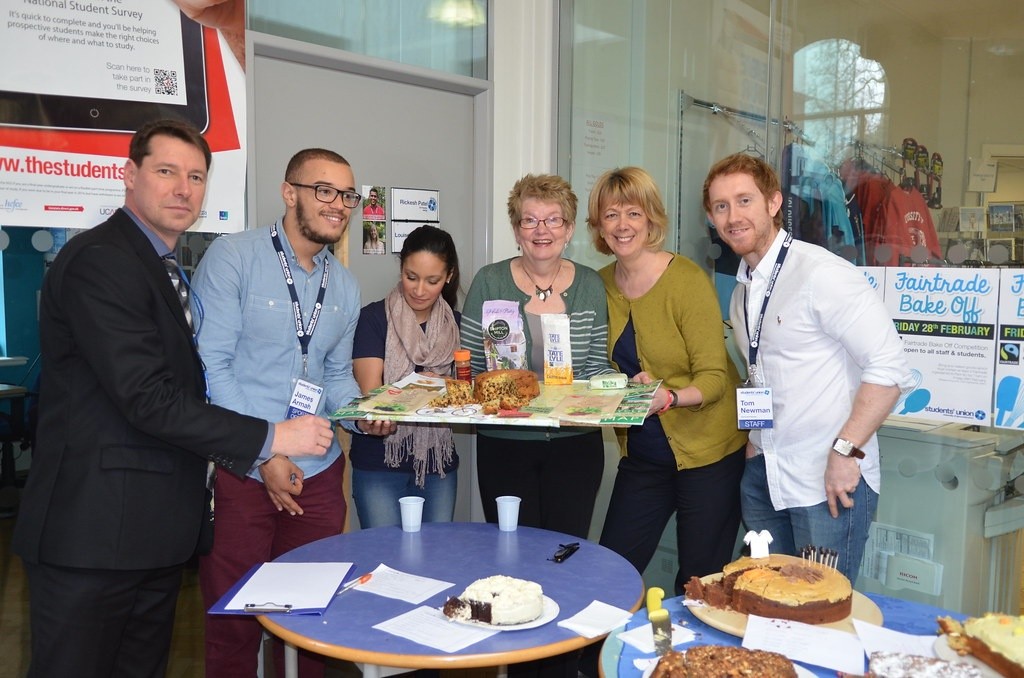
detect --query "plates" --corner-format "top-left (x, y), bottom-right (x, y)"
top-left (685, 572), bottom-right (883, 636)
top-left (935, 631), bottom-right (1005, 677)
top-left (454, 595), bottom-right (559, 631)
top-left (643, 651), bottom-right (819, 678)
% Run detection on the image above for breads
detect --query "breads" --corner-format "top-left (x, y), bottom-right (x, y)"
top-left (647, 644), bottom-right (800, 678)
top-left (430, 370), bottom-right (542, 415)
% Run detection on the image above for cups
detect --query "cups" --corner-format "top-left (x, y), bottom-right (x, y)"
top-left (495, 496), bottom-right (521, 531)
top-left (399, 496), bottom-right (425, 532)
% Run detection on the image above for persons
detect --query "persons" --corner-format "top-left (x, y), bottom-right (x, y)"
top-left (586, 167), bottom-right (750, 594)
top-left (704, 155), bottom-right (919, 590)
top-left (352, 226), bottom-right (462, 529)
top-left (189, 149), bottom-right (396, 678)
top-left (460, 175), bottom-right (610, 539)
top-left (27, 122), bottom-right (332, 678)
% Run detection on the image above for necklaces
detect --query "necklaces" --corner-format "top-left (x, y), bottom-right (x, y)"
top-left (522, 263), bottom-right (562, 302)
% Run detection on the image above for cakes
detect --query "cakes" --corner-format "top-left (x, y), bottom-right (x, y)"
top-left (937, 612), bottom-right (1024, 678)
top-left (444, 575), bottom-right (543, 625)
top-left (685, 554), bottom-right (853, 624)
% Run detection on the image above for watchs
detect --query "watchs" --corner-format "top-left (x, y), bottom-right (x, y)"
top-left (832, 438), bottom-right (865, 460)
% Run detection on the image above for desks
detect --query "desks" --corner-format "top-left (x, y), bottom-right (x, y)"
top-left (255, 522), bottom-right (646, 678)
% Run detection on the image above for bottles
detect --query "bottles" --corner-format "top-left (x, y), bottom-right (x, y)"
top-left (453, 349), bottom-right (473, 389)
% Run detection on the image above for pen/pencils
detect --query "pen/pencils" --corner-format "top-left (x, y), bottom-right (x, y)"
top-left (337, 573), bottom-right (373, 597)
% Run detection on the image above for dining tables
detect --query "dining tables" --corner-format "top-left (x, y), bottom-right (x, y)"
top-left (599, 589), bottom-right (1004, 678)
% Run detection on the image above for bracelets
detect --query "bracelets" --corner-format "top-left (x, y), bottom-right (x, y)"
top-left (355, 421), bottom-right (369, 434)
top-left (658, 390), bottom-right (672, 415)
top-left (669, 389), bottom-right (678, 409)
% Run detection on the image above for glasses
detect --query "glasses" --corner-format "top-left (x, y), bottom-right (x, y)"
top-left (290, 182), bottom-right (362, 208)
top-left (519, 216), bottom-right (567, 229)
top-left (161, 259), bottom-right (200, 354)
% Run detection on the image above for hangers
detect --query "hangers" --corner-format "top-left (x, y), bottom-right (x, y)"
top-left (741, 129), bottom-right (763, 156)
top-left (789, 123), bottom-right (913, 193)
